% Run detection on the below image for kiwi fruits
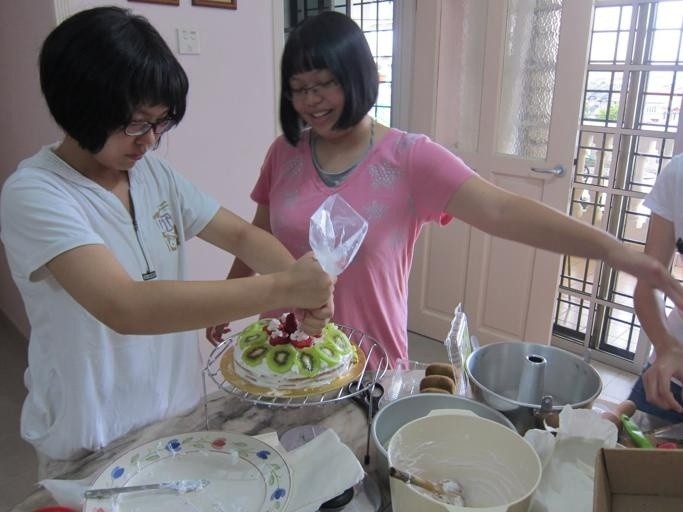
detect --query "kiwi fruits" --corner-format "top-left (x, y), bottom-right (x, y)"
top-left (419, 362), bottom-right (457, 394)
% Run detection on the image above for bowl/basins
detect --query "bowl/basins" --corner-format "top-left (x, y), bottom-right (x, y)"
top-left (388, 407), bottom-right (543, 512)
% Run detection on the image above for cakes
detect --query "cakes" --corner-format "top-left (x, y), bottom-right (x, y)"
top-left (232, 310), bottom-right (357, 391)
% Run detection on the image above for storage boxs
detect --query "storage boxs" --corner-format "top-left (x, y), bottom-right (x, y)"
top-left (591, 447), bottom-right (683, 511)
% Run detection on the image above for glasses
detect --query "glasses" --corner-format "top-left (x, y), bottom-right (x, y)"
top-left (287, 78), bottom-right (338, 101)
top-left (123, 115), bottom-right (177, 135)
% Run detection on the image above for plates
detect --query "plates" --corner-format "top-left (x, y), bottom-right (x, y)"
top-left (80, 431), bottom-right (292, 512)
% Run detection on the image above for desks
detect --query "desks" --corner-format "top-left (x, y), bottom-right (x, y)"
top-left (11, 371), bottom-right (682, 512)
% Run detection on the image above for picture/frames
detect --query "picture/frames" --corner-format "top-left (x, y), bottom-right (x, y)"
top-left (191, 0), bottom-right (237, 10)
top-left (128, 0), bottom-right (181, 6)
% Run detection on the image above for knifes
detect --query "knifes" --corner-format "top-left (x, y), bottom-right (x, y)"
top-left (84, 480), bottom-right (207, 499)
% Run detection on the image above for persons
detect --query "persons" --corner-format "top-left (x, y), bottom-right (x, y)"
top-left (0, 6), bottom-right (337, 486)
top-left (626, 151), bottom-right (682, 425)
top-left (204, 13), bottom-right (682, 372)
